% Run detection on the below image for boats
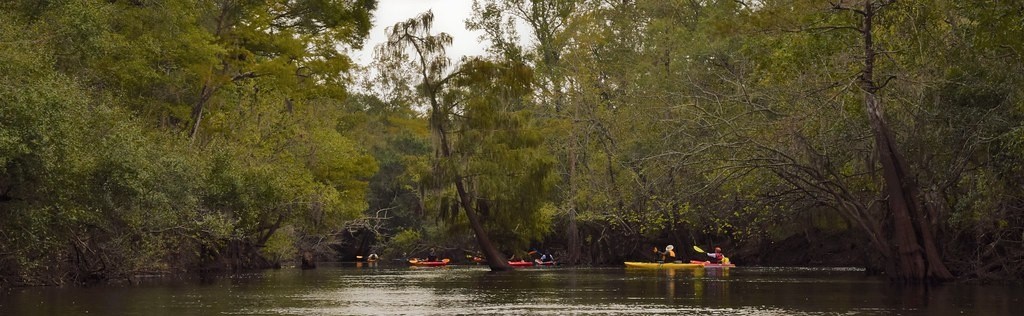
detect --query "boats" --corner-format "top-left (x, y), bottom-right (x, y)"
top-left (409, 258), bottom-right (450, 266)
top-left (689, 260), bottom-right (736, 268)
top-left (535, 258), bottom-right (555, 265)
top-left (507, 260), bottom-right (533, 267)
top-left (624, 261), bottom-right (705, 270)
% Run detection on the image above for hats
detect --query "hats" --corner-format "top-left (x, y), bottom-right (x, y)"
top-left (666, 245), bottom-right (673, 250)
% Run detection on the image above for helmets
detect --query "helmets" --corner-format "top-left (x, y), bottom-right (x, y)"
top-left (715, 248), bottom-right (721, 251)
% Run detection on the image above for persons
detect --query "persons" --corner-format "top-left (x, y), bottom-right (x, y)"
top-left (540, 250), bottom-right (554, 262)
top-left (367, 250), bottom-right (379, 260)
top-left (707, 247), bottom-right (725, 264)
top-left (510, 254), bottom-right (523, 262)
top-left (428, 248), bottom-right (438, 261)
top-left (659, 245), bottom-right (678, 264)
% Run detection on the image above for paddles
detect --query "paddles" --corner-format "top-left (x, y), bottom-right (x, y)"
top-left (694, 245), bottom-right (706, 253)
